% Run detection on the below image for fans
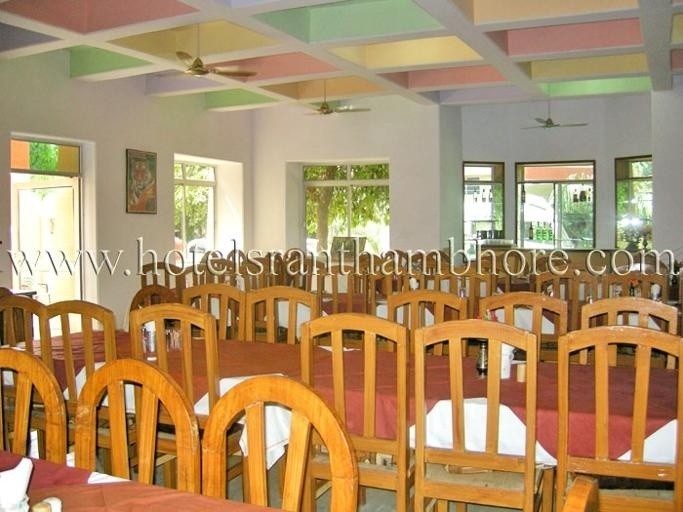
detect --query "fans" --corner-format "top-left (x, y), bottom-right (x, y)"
top-left (291, 78), bottom-right (370, 117)
top-left (151, 22), bottom-right (258, 80)
top-left (520, 97), bottom-right (590, 130)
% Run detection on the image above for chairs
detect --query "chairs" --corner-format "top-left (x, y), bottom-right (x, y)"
top-left (0, 245), bottom-right (682, 512)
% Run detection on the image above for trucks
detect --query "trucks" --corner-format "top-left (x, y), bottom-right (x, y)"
top-left (463, 193), bottom-right (574, 261)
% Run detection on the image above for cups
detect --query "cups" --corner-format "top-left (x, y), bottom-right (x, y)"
top-left (165, 329), bottom-right (182, 352)
top-left (515, 361), bottom-right (524, 381)
top-left (475, 230), bottom-right (504, 239)
top-left (500, 350), bottom-right (510, 380)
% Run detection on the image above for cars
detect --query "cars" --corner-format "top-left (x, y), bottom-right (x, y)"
top-left (173, 234), bottom-right (204, 262)
top-left (305, 237), bottom-right (330, 259)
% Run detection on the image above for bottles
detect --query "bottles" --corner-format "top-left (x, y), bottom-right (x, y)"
top-left (142, 323), bottom-right (157, 361)
top-left (670, 278), bottom-right (678, 300)
top-left (528, 220), bottom-right (553, 241)
top-left (573, 182), bottom-right (591, 202)
top-left (476, 340), bottom-right (488, 377)
top-left (473, 187), bottom-right (493, 203)
top-left (521, 185), bottom-right (525, 202)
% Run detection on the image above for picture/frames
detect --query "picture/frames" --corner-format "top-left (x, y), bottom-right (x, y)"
top-left (124, 147), bottom-right (158, 215)
